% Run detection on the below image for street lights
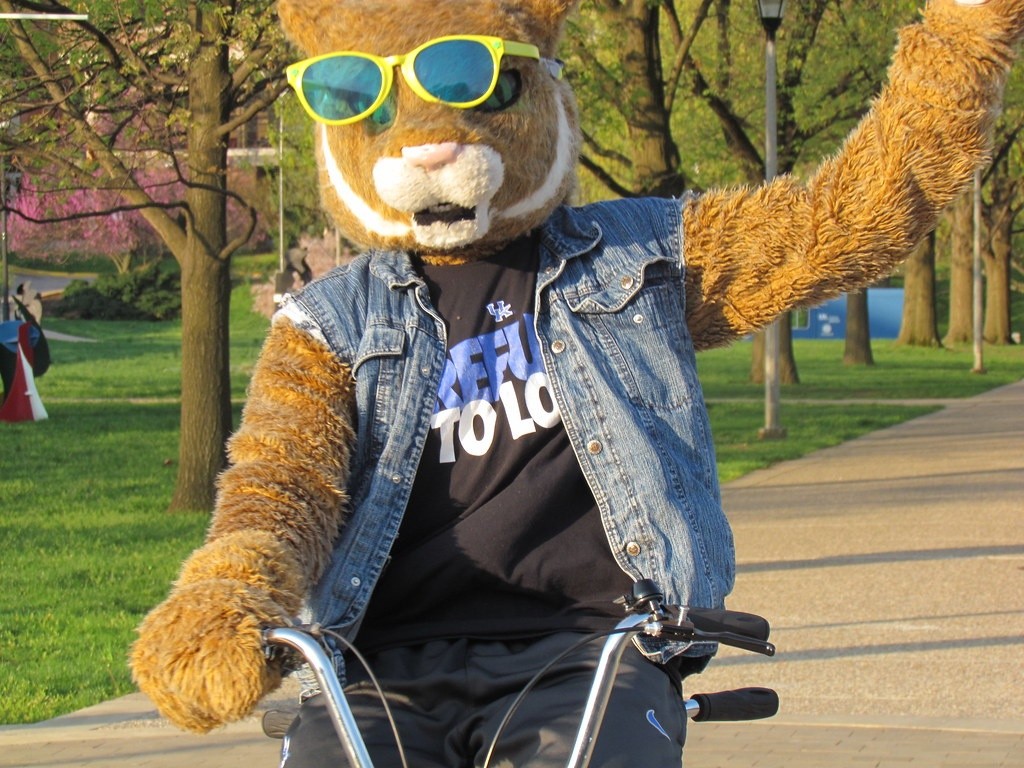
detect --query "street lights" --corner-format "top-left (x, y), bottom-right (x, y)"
top-left (750, 0), bottom-right (788, 441)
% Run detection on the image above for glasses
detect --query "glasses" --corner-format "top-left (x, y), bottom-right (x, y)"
top-left (285, 34), bottom-right (540, 126)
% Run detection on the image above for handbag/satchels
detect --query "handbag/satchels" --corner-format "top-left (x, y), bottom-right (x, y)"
top-left (0, 320), bottom-right (50, 376)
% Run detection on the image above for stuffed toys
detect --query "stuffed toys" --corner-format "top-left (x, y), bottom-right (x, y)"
top-left (129, 1), bottom-right (1024, 768)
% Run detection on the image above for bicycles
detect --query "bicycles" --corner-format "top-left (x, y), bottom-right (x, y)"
top-left (255, 579), bottom-right (778, 768)
